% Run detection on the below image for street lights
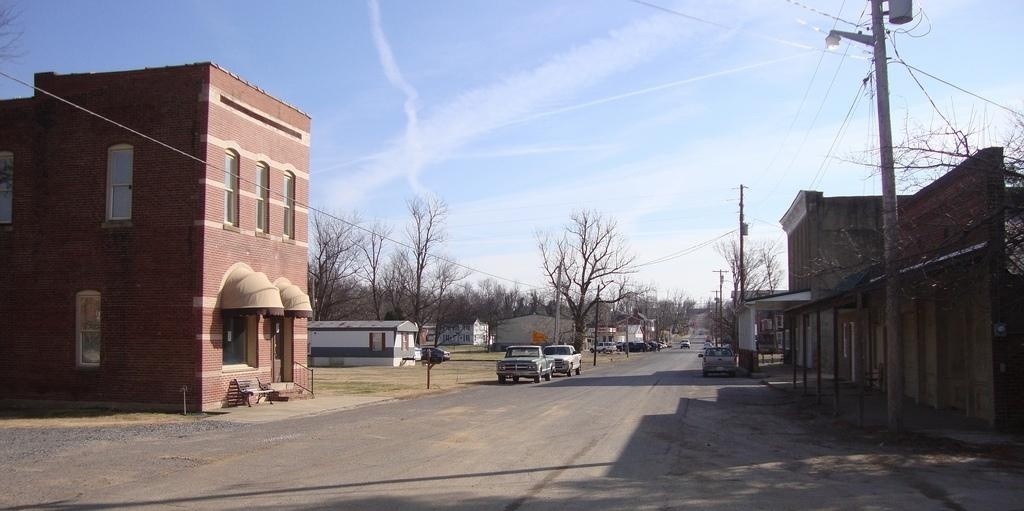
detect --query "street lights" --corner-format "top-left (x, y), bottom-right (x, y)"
top-left (826, 29), bottom-right (905, 431)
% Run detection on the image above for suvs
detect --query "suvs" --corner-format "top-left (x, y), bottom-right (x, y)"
top-left (590, 342), bottom-right (617, 354)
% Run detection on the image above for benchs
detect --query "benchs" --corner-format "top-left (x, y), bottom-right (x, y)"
top-left (232, 377), bottom-right (276, 408)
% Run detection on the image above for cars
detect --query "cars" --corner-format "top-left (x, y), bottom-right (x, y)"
top-left (421, 347), bottom-right (450, 364)
top-left (680, 339), bottom-right (691, 349)
top-left (617, 340), bottom-right (668, 352)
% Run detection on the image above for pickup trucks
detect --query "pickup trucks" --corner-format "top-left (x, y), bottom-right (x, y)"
top-left (496, 346), bottom-right (555, 383)
top-left (544, 344), bottom-right (582, 376)
top-left (698, 347), bottom-right (738, 377)
